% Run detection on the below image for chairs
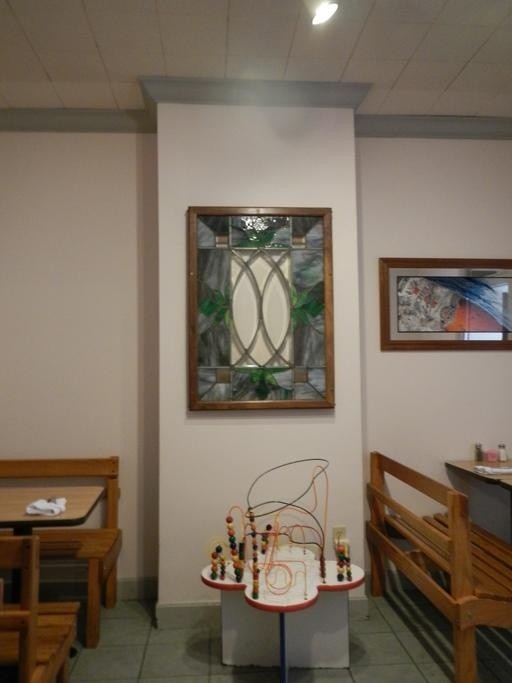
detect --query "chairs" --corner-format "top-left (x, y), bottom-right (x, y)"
top-left (0, 534), bottom-right (81, 683)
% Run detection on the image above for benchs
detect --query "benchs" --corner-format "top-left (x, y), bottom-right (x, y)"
top-left (365, 452), bottom-right (510, 682)
top-left (0, 456), bottom-right (121, 609)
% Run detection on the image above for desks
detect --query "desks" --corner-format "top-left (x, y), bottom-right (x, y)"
top-left (0, 484), bottom-right (106, 648)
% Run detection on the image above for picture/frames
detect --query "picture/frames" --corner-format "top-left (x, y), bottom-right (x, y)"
top-left (186, 204), bottom-right (334, 410)
top-left (378, 257), bottom-right (512, 351)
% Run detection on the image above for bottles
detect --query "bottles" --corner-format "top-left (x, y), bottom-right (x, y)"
top-left (498, 444), bottom-right (507, 463)
top-left (475, 443), bottom-right (483, 462)
